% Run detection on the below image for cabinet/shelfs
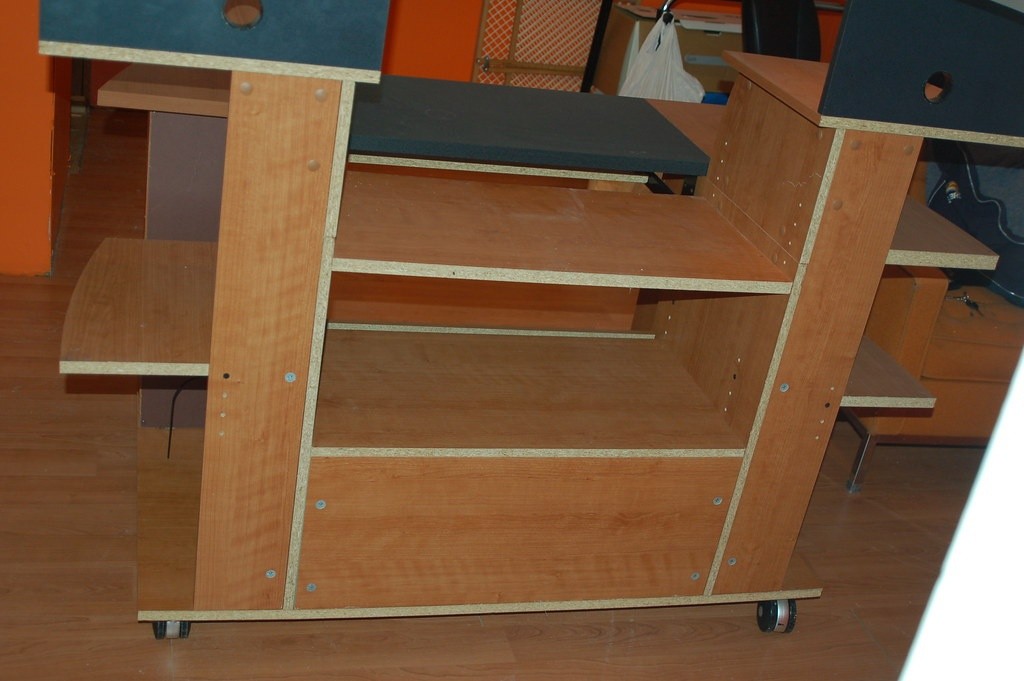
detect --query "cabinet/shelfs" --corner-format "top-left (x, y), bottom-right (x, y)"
top-left (39, 0), bottom-right (1024, 639)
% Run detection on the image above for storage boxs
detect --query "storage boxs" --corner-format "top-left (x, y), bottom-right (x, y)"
top-left (593, 3), bottom-right (739, 96)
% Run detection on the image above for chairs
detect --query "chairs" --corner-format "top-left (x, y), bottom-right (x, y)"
top-left (741, 0), bottom-right (821, 62)
top-left (834, 135), bottom-right (1024, 493)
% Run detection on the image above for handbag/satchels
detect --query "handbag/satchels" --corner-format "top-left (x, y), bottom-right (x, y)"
top-left (619, 13), bottom-right (705, 103)
